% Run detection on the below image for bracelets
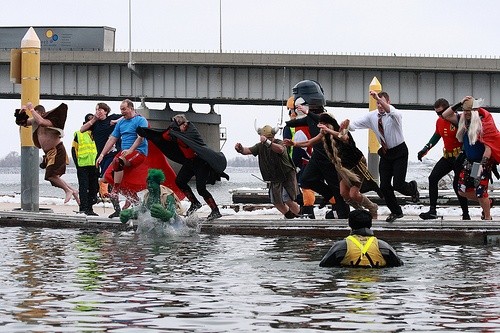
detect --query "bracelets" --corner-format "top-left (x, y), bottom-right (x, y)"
top-left (29, 108), bottom-right (34, 112)
top-left (264, 139), bottom-right (272, 147)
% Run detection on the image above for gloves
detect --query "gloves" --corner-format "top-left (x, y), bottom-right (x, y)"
top-left (417, 151), bottom-right (424, 162)
top-left (119, 208), bottom-right (137, 224)
top-left (150, 204), bottom-right (172, 222)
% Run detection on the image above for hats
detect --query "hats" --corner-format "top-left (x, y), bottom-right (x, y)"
top-left (461, 96), bottom-right (480, 111)
top-left (348, 209), bottom-right (373, 230)
top-left (257, 125), bottom-right (276, 138)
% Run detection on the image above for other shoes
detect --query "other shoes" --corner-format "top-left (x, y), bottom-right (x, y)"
top-left (185, 202), bottom-right (202, 216)
top-left (207, 212), bottom-right (222, 221)
top-left (87, 211), bottom-right (99, 216)
top-left (319, 192), bottom-right (334, 209)
top-left (419, 207), bottom-right (437, 219)
top-left (480, 197), bottom-right (495, 220)
top-left (409, 180), bottom-right (419, 203)
top-left (386, 206), bottom-right (403, 222)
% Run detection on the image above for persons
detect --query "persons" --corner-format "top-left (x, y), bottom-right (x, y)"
top-left (320, 210), bottom-right (403, 268)
top-left (282, 79), bottom-right (383, 219)
top-left (340, 90), bottom-right (419, 222)
top-left (418, 98), bottom-right (470, 219)
top-left (136, 115), bottom-right (229, 220)
top-left (72, 113), bottom-right (112, 216)
top-left (14, 102), bottom-right (81, 204)
top-left (119, 169), bottom-right (176, 226)
top-left (96, 100), bottom-right (186, 210)
top-left (234, 126), bottom-right (303, 218)
top-left (80, 103), bottom-right (120, 218)
top-left (442, 96), bottom-right (500, 220)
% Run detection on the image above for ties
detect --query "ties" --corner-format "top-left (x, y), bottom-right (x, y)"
top-left (378, 116), bottom-right (387, 154)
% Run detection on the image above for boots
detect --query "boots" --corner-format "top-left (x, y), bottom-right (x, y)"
top-left (360, 194), bottom-right (378, 217)
top-left (108, 200), bottom-right (122, 218)
top-left (344, 198), bottom-right (364, 210)
